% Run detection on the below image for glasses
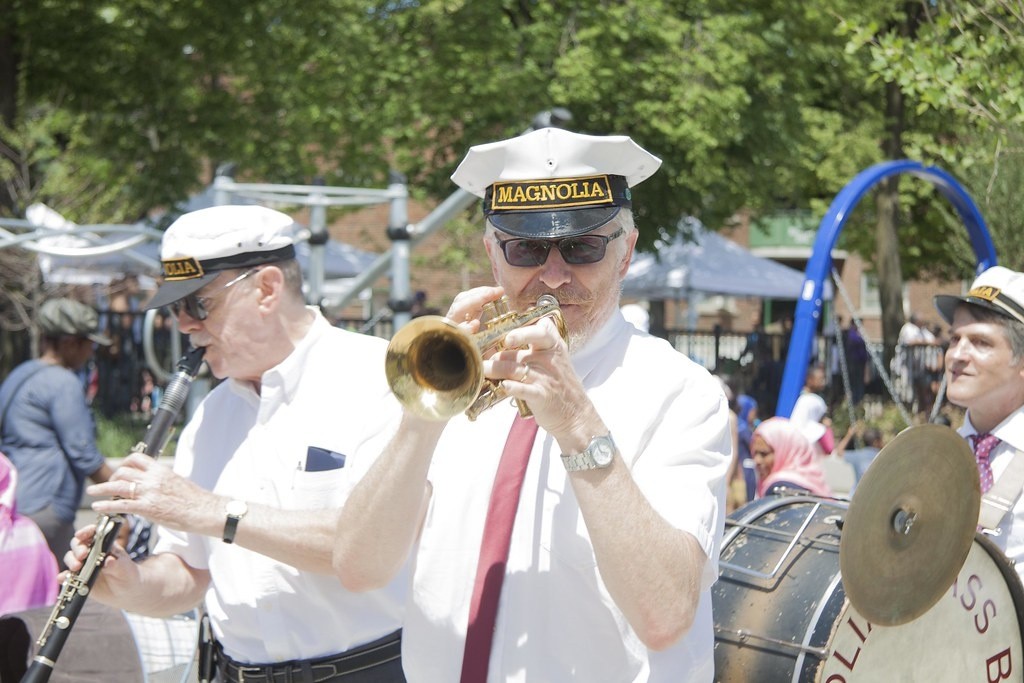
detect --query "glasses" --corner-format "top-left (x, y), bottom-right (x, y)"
top-left (493, 228), bottom-right (624, 266)
top-left (170, 270), bottom-right (259, 320)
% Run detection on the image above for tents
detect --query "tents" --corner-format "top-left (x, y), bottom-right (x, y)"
top-left (619, 213), bottom-right (834, 369)
top-left (135, 170), bottom-right (397, 281)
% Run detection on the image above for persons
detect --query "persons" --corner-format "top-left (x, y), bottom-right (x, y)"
top-left (888, 309), bottom-right (945, 410)
top-left (0, 272), bottom-right (183, 619)
top-left (933, 265), bottom-right (1024, 586)
top-left (332, 128), bottom-right (734, 683)
top-left (830, 315), bottom-right (868, 398)
top-left (720, 364), bottom-right (856, 511)
top-left (60, 205), bottom-right (419, 683)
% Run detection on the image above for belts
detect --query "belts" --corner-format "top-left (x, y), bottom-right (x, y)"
top-left (215, 638), bottom-right (401, 683)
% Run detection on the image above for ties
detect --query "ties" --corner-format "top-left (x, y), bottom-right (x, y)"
top-left (460, 411), bottom-right (540, 683)
top-left (969, 433), bottom-right (1001, 494)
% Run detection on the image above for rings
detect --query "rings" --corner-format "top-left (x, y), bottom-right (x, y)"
top-left (521, 364), bottom-right (529, 382)
top-left (129, 482), bottom-right (137, 498)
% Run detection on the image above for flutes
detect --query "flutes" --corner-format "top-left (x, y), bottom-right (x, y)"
top-left (17, 346), bottom-right (209, 683)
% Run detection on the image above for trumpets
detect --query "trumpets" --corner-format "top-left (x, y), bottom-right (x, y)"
top-left (384, 289), bottom-right (561, 424)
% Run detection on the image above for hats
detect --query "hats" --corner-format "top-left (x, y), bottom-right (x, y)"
top-left (37, 298), bottom-right (114, 346)
top-left (450, 126), bottom-right (663, 240)
top-left (933, 266), bottom-right (1024, 325)
top-left (143, 204), bottom-right (296, 312)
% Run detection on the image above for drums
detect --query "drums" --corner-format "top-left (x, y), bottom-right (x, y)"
top-left (711, 480), bottom-right (1024, 683)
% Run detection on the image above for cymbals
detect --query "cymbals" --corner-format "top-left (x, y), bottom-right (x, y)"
top-left (837, 417), bottom-right (984, 629)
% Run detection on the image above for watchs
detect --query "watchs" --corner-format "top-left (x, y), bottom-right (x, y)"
top-left (561, 430), bottom-right (618, 473)
top-left (223, 496), bottom-right (249, 545)
